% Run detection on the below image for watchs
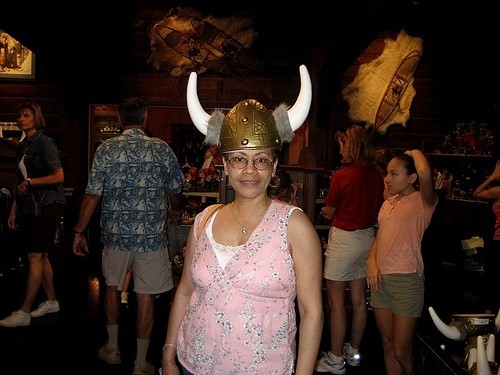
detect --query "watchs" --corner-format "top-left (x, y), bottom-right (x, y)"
top-left (25, 178), bottom-right (32, 186)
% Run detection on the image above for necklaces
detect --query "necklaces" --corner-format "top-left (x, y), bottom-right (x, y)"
top-left (231, 197), bottom-right (270, 236)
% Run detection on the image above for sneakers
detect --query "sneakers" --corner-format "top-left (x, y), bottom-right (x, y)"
top-left (30, 300), bottom-right (59, 317)
top-left (342, 343), bottom-right (361, 366)
top-left (120, 292), bottom-right (128, 304)
top-left (133, 361), bottom-right (155, 375)
top-left (98, 343), bottom-right (122, 364)
top-left (0, 308), bottom-right (31, 327)
top-left (314, 351), bottom-right (346, 374)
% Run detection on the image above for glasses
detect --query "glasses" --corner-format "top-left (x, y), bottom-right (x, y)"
top-left (224, 155), bottom-right (275, 170)
top-left (341, 127), bottom-right (348, 140)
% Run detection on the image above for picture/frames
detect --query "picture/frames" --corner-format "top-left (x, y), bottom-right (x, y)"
top-left (0, 28), bottom-right (36, 80)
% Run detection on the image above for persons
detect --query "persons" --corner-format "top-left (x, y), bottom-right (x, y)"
top-left (162, 99), bottom-right (324, 375)
top-left (366, 149), bottom-right (439, 375)
top-left (0, 102), bottom-right (64, 327)
top-left (72, 95), bottom-right (185, 375)
top-left (267, 169), bottom-right (304, 209)
top-left (472, 159), bottom-right (500, 337)
top-left (314, 123), bottom-right (385, 375)
top-left (198, 143), bottom-right (225, 203)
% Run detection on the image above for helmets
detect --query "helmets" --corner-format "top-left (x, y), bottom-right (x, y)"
top-left (219, 99), bottom-right (281, 152)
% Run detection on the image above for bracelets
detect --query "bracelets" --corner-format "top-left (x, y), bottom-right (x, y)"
top-left (163, 344), bottom-right (177, 356)
top-left (74, 228), bottom-right (84, 235)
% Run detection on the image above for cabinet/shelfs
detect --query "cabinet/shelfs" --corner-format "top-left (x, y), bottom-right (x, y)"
top-left (169, 153), bottom-right (495, 313)
